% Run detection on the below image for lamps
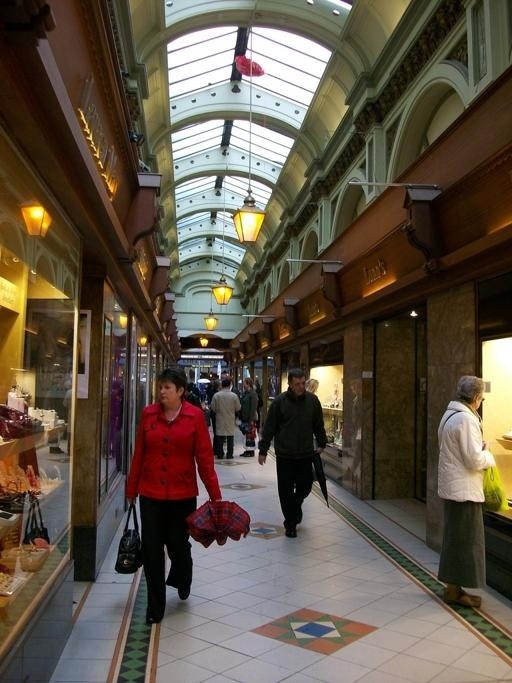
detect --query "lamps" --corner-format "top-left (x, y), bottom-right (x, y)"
top-left (211, 275), bottom-right (235, 306)
top-left (138, 333), bottom-right (148, 346)
top-left (203, 310), bottom-right (220, 332)
top-left (198, 338), bottom-right (209, 347)
top-left (22, 201), bottom-right (55, 237)
top-left (119, 314), bottom-right (132, 330)
top-left (232, 196), bottom-right (270, 242)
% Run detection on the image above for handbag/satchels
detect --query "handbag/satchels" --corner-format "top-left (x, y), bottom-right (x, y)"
top-left (19, 525), bottom-right (52, 575)
top-left (244, 431), bottom-right (260, 451)
top-left (113, 528), bottom-right (142, 575)
top-left (27, 406), bottom-right (58, 430)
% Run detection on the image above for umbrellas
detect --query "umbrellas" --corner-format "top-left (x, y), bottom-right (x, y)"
top-left (183, 498), bottom-right (251, 548)
top-left (309, 445), bottom-right (330, 507)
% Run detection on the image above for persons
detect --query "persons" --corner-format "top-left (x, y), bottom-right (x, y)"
top-left (305, 376), bottom-right (319, 395)
top-left (438, 376), bottom-right (496, 608)
top-left (125, 367), bottom-right (221, 623)
top-left (186, 368), bottom-right (262, 459)
top-left (40, 365), bottom-right (71, 454)
top-left (100, 373), bottom-right (126, 458)
top-left (258, 369), bottom-right (329, 538)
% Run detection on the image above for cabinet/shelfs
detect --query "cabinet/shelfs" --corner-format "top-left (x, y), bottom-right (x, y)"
top-left (1, 402), bottom-right (68, 613)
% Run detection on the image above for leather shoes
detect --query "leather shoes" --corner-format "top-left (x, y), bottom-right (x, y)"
top-left (177, 574), bottom-right (192, 602)
top-left (293, 499), bottom-right (304, 525)
top-left (443, 586), bottom-right (483, 609)
top-left (146, 598), bottom-right (166, 624)
top-left (284, 522), bottom-right (297, 538)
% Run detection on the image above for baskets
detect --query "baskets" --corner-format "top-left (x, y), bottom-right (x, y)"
top-left (2, 542), bottom-right (51, 573)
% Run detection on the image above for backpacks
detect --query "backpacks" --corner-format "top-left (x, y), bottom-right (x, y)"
top-left (0, 401), bottom-right (45, 440)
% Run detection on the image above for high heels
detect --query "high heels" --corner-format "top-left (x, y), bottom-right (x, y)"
top-left (241, 450), bottom-right (257, 456)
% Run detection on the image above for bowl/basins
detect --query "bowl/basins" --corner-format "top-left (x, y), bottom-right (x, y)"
top-left (496, 438), bottom-right (512, 452)
